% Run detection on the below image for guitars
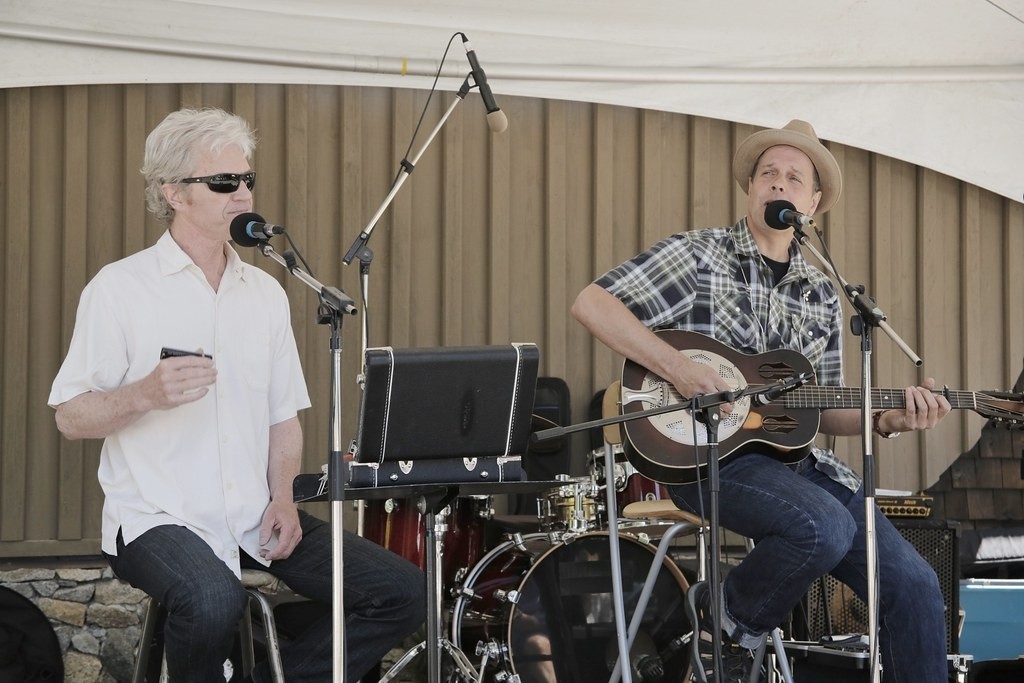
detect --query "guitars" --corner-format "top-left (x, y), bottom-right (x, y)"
top-left (617, 328), bottom-right (1023, 487)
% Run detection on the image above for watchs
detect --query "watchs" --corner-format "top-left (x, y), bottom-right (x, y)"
top-left (874, 410), bottom-right (900, 439)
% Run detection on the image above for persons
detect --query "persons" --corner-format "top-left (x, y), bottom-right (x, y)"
top-left (571, 120), bottom-right (952, 683)
top-left (47, 109), bottom-right (428, 683)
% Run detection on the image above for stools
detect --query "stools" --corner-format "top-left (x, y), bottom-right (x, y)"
top-left (114, 569), bottom-right (287, 683)
top-left (604, 379), bottom-right (794, 683)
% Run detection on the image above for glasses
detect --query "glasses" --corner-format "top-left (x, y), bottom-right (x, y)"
top-left (156, 171), bottom-right (261, 193)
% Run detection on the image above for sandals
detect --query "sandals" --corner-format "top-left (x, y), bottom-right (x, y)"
top-left (685, 581), bottom-right (752, 682)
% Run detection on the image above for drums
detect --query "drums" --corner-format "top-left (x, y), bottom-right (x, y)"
top-left (452, 527), bottom-right (699, 682)
top-left (361, 491), bottom-right (494, 603)
top-left (539, 473), bottom-right (612, 533)
top-left (586, 440), bottom-right (700, 539)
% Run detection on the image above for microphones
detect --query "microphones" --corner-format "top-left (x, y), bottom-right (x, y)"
top-left (764, 199), bottom-right (815, 231)
top-left (460, 32), bottom-right (508, 133)
top-left (229, 213), bottom-right (286, 247)
top-left (750, 371), bottom-right (816, 408)
top-left (604, 631), bottom-right (665, 683)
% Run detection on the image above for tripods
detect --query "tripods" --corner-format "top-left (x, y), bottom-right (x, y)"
top-left (377, 499), bottom-right (482, 683)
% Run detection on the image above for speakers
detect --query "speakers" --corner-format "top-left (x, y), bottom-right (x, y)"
top-left (793, 516), bottom-right (963, 683)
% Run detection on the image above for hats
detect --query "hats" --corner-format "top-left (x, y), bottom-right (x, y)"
top-left (732, 119), bottom-right (842, 216)
